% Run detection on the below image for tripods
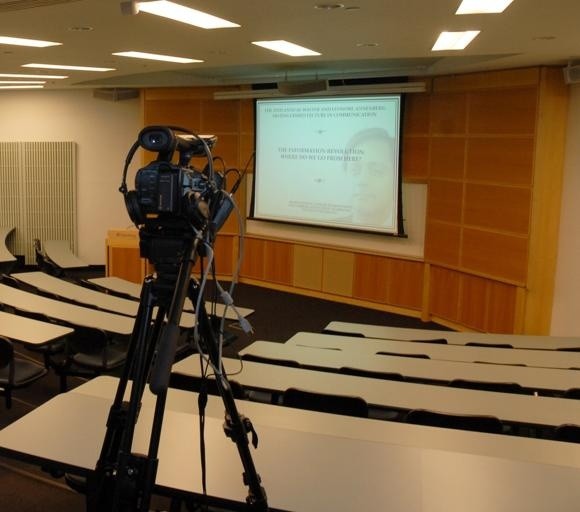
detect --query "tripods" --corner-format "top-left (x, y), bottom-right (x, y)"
top-left (79, 275), bottom-right (271, 510)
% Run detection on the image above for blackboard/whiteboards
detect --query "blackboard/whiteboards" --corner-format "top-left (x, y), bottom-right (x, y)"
top-left (243, 168), bottom-right (431, 260)
